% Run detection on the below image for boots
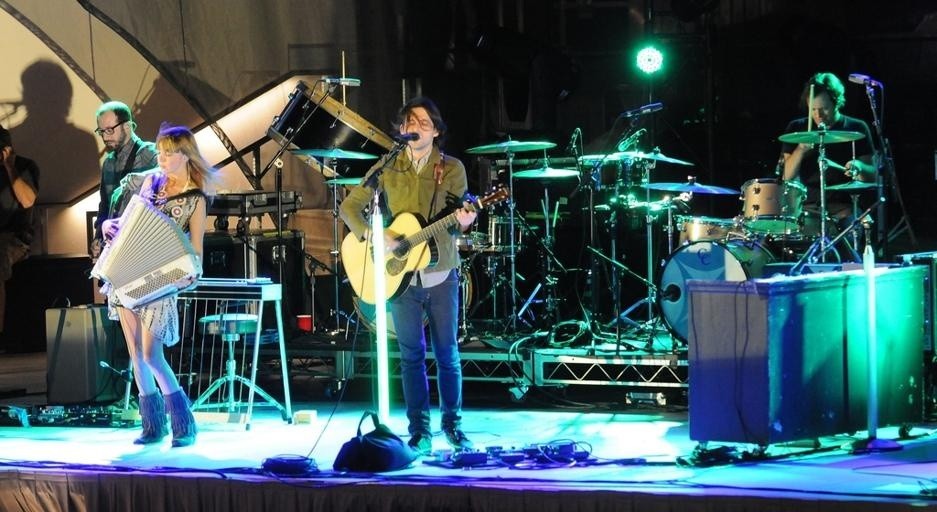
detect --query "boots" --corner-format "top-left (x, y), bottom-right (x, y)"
top-left (133, 386), bottom-right (197, 447)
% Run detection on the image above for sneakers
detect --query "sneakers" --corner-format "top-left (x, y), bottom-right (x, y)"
top-left (441, 428), bottom-right (474, 456)
top-left (405, 436), bottom-right (432, 456)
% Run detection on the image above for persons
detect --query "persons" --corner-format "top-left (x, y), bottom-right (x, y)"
top-left (101, 123), bottom-right (208, 446)
top-left (0, 126), bottom-right (38, 322)
top-left (89, 99), bottom-right (160, 419)
top-left (777, 68), bottom-right (875, 257)
top-left (335, 96), bottom-right (478, 455)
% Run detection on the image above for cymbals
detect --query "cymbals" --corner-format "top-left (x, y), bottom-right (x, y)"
top-left (512, 168), bottom-right (581, 179)
top-left (622, 152), bottom-right (694, 168)
top-left (780, 130), bottom-right (864, 147)
top-left (466, 142), bottom-right (556, 155)
top-left (643, 182), bottom-right (740, 196)
top-left (824, 181), bottom-right (880, 190)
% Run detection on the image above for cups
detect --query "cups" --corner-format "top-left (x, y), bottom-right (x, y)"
top-left (296, 315), bottom-right (312, 332)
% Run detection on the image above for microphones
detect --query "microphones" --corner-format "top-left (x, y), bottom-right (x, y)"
top-left (848, 71), bottom-right (877, 89)
top-left (565, 128), bottom-right (580, 154)
top-left (325, 77), bottom-right (361, 88)
top-left (396, 132), bottom-right (420, 142)
top-left (622, 102), bottom-right (664, 118)
top-left (617, 128), bottom-right (648, 151)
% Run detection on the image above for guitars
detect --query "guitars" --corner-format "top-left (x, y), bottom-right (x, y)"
top-left (341, 187), bottom-right (506, 305)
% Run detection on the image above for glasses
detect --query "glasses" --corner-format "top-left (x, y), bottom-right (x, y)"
top-left (95, 121), bottom-right (124, 136)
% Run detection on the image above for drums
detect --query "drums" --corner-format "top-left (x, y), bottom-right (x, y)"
top-left (742, 178), bottom-right (806, 234)
top-left (351, 258), bottom-right (479, 340)
top-left (269, 81), bottom-right (399, 193)
top-left (654, 238), bottom-right (775, 345)
top-left (791, 209), bottom-right (835, 247)
top-left (679, 215), bottom-right (742, 247)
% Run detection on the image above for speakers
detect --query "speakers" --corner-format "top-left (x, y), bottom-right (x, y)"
top-left (44, 304), bottom-right (133, 405)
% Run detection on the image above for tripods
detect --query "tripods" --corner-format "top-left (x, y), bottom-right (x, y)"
top-left (458, 176), bottom-right (666, 352)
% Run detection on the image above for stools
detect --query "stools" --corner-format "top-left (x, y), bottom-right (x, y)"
top-left (189, 314), bottom-right (288, 426)
